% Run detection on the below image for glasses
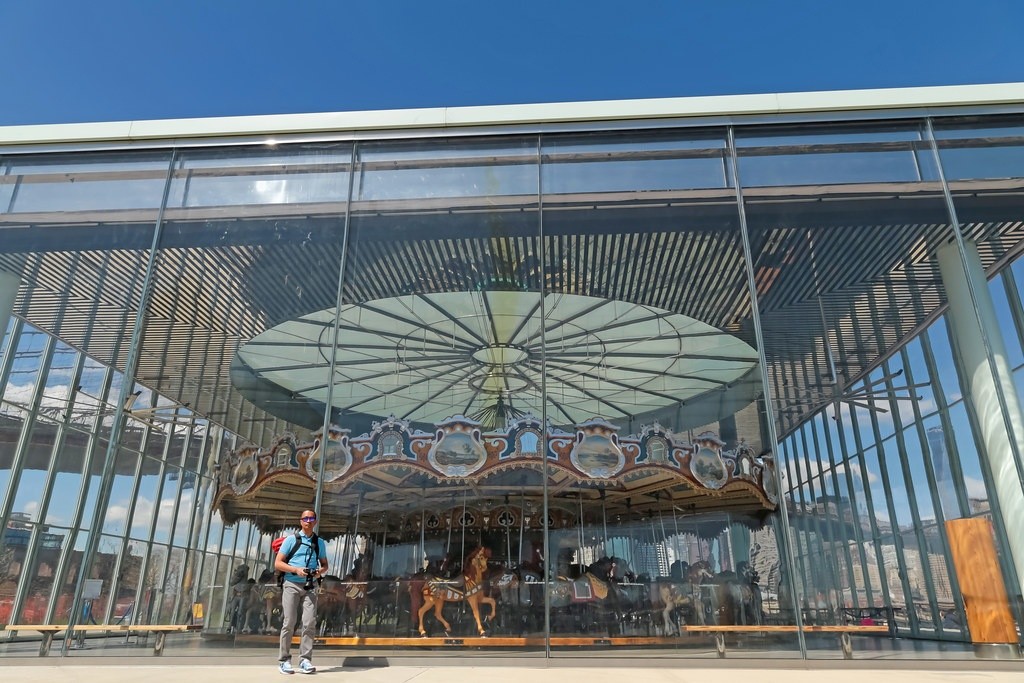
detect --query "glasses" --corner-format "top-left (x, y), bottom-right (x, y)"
top-left (301, 517), bottom-right (316, 522)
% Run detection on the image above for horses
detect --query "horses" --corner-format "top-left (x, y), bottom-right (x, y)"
top-left (225, 546), bottom-right (762, 637)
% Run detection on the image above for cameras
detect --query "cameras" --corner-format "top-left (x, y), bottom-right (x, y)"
top-left (303, 568), bottom-right (316, 590)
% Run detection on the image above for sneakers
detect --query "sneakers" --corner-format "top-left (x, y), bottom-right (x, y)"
top-left (278, 659), bottom-right (294, 674)
top-left (298, 657), bottom-right (316, 673)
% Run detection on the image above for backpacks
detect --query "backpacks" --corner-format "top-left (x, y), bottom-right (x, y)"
top-left (271, 532), bottom-right (320, 590)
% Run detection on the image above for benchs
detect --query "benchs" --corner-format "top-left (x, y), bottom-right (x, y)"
top-left (681, 625), bottom-right (888, 660)
top-left (5, 624), bottom-right (204, 656)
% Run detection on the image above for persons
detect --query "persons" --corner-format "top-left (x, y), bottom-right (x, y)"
top-left (274, 509), bottom-right (328, 675)
top-left (940, 608), bottom-right (960, 629)
